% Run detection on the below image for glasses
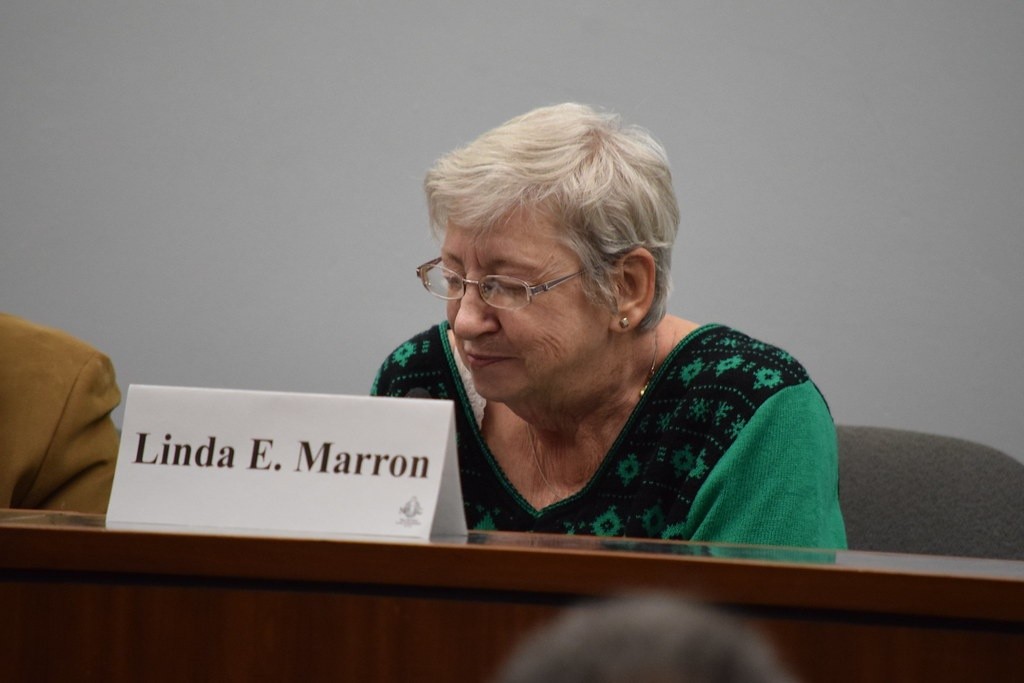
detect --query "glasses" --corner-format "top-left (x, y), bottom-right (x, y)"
top-left (416, 256), bottom-right (605, 310)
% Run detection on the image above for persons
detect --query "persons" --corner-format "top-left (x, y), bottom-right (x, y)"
top-left (373, 101), bottom-right (847, 549)
top-left (0, 315), bottom-right (119, 514)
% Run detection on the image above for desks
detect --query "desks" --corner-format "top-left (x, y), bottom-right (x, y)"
top-left (0, 505), bottom-right (1024, 683)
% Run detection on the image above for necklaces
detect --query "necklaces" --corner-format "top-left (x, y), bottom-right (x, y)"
top-left (526, 326), bottom-right (658, 500)
